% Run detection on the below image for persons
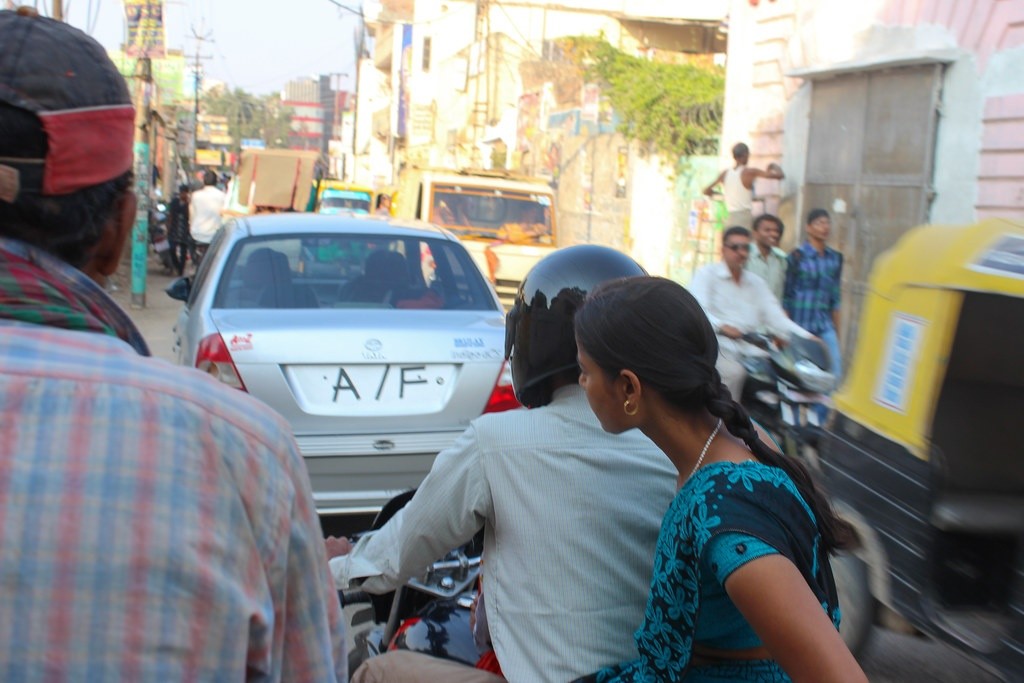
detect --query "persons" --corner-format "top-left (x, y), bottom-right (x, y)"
top-left (567, 277), bottom-right (870, 683)
top-left (687, 227), bottom-right (789, 419)
top-left (703, 142), bottom-right (784, 235)
top-left (187, 171), bottom-right (229, 265)
top-left (167, 184), bottom-right (190, 277)
top-left (780, 209), bottom-right (844, 427)
top-left (0, 6), bottom-right (349, 683)
top-left (322, 245), bottom-right (682, 683)
top-left (513, 204), bottom-right (548, 246)
top-left (743, 214), bottom-right (789, 337)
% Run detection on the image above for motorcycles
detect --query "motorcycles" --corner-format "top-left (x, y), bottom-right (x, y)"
top-left (816, 221), bottom-right (1023, 683)
top-left (338, 488), bottom-right (491, 672)
top-left (738, 333), bottom-right (835, 464)
top-left (149, 202), bottom-right (175, 272)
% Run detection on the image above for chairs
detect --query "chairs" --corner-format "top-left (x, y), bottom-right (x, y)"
top-left (233, 246), bottom-right (421, 310)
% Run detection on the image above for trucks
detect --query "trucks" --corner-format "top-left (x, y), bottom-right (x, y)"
top-left (308, 181), bottom-right (378, 214)
top-left (394, 166), bottom-right (559, 308)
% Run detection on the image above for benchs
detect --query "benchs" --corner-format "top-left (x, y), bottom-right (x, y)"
top-left (935, 492), bottom-right (1023, 537)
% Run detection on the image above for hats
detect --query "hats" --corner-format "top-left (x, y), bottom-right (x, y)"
top-left (0, 8), bottom-right (135, 195)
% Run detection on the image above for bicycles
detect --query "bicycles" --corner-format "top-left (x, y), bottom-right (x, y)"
top-left (191, 242), bottom-right (208, 270)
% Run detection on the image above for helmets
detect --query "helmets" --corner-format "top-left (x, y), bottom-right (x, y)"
top-left (505, 244), bottom-right (652, 408)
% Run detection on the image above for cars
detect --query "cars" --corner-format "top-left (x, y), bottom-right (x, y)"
top-left (298, 233), bottom-right (388, 277)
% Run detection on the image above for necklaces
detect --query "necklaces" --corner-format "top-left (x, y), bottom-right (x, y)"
top-left (684, 418), bottom-right (724, 484)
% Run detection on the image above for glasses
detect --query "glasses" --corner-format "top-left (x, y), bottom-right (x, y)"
top-left (726, 242), bottom-right (752, 253)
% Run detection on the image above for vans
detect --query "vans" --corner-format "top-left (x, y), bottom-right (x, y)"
top-left (165, 213), bottom-right (527, 516)
